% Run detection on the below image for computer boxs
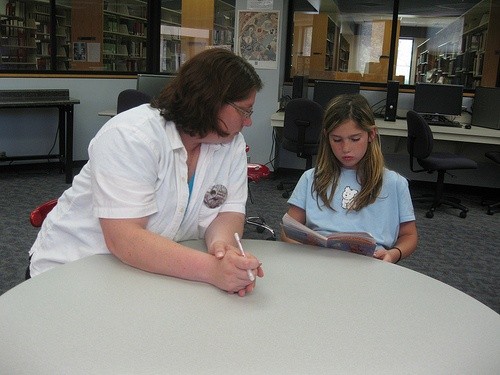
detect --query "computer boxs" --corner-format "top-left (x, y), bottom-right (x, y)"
top-left (292, 75), bottom-right (308, 100)
top-left (385, 81), bottom-right (400, 121)
top-left (471, 86), bottom-right (500, 130)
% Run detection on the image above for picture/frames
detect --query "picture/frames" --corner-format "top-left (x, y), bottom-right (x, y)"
top-left (239, 9), bottom-right (281, 71)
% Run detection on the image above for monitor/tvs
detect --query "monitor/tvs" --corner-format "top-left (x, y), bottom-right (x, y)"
top-left (136, 73), bottom-right (177, 98)
top-left (312, 80), bottom-right (361, 108)
top-left (414, 82), bottom-right (464, 120)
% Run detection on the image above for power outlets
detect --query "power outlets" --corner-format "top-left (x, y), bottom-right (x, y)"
top-left (0, 152), bottom-right (6, 160)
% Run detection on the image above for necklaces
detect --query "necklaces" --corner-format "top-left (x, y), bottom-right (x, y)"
top-left (186, 154), bottom-right (194, 166)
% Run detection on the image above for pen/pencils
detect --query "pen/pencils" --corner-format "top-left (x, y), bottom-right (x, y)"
top-left (234, 232), bottom-right (255, 281)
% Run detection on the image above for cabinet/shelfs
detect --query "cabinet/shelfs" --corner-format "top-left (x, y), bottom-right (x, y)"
top-left (159, 7), bottom-right (183, 72)
top-left (180, 0), bottom-right (236, 70)
top-left (32, 1), bottom-right (72, 71)
top-left (72, 0), bottom-right (149, 70)
top-left (413, 0), bottom-right (500, 86)
top-left (0, 24), bottom-right (36, 65)
top-left (290, 12), bottom-right (348, 74)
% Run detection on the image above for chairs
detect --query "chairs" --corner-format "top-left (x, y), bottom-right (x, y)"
top-left (244, 145), bottom-right (274, 238)
top-left (276, 97), bottom-right (324, 199)
top-left (401, 110), bottom-right (500, 219)
top-left (117, 89), bottom-right (150, 116)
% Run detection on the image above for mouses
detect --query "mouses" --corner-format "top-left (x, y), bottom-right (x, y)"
top-left (465, 124), bottom-right (471, 129)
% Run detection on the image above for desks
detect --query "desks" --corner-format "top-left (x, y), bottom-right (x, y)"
top-left (0, 90), bottom-right (80, 185)
top-left (0, 237), bottom-right (499, 375)
top-left (269, 111), bottom-right (500, 144)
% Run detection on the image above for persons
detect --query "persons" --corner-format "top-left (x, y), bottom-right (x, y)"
top-left (281, 93), bottom-right (418, 263)
top-left (25, 46), bottom-right (263, 296)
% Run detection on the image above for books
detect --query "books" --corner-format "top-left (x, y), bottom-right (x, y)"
top-left (103, 0), bottom-right (182, 72)
top-left (0, 0), bottom-right (71, 68)
top-left (416, 14), bottom-right (489, 88)
top-left (325, 31), bottom-right (347, 72)
top-left (214, 28), bottom-right (233, 52)
top-left (281, 212), bottom-right (377, 256)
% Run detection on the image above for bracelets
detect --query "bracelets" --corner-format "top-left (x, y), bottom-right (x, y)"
top-left (390, 247), bottom-right (402, 264)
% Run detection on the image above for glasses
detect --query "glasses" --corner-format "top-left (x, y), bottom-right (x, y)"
top-left (224, 99), bottom-right (254, 121)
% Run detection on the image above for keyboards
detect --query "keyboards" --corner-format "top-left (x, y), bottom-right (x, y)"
top-left (426, 119), bottom-right (461, 127)
